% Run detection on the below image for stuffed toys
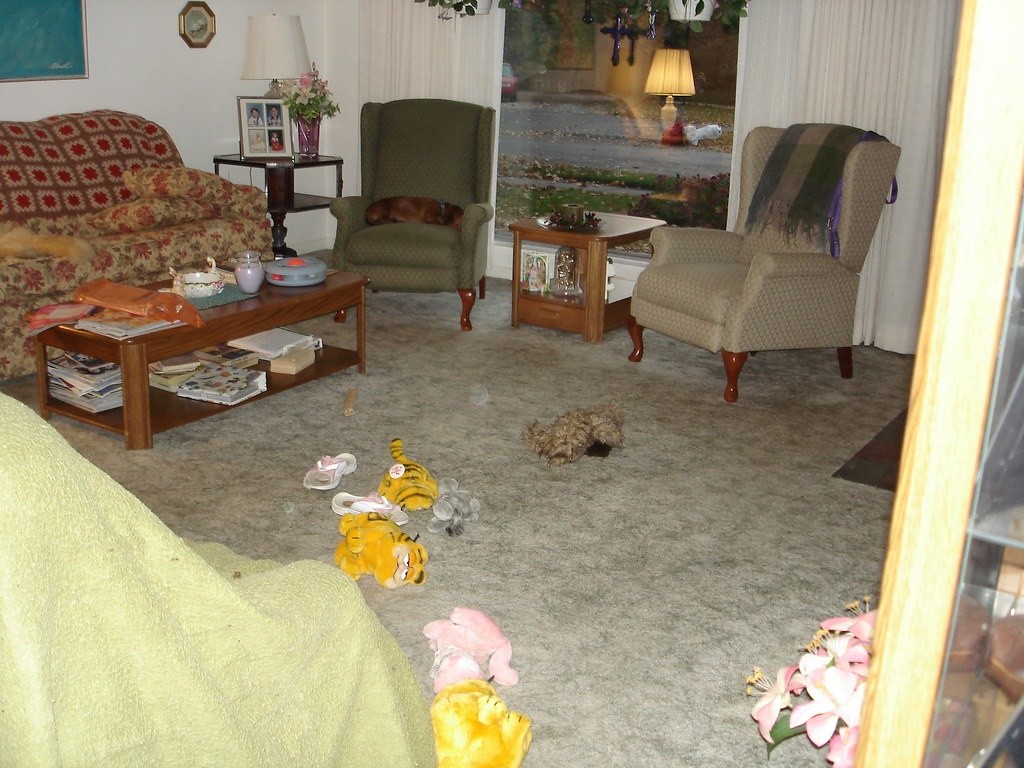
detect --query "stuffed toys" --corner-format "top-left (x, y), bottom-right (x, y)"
top-left (335, 511), bottom-right (428, 589)
top-left (525, 403), bottom-right (625, 469)
top-left (377, 438), bottom-right (438, 512)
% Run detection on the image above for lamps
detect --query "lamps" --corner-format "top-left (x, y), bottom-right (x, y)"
top-left (644, 48), bottom-right (695, 131)
top-left (241, 14), bottom-right (313, 98)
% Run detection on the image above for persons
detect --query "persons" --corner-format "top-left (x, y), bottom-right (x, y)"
top-left (249, 106), bottom-right (283, 151)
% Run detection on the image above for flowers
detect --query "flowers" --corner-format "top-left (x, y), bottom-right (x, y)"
top-left (273, 61), bottom-right (341, 125)
top-left (619, 0), bottom-right (747, 33)
top-left (747, 597), bottom-right (876, 768)
top-left (415, 0), bottom-right (522, 20)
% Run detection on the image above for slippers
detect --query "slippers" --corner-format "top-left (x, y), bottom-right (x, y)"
top-left (332, 491), bottom-right (409, 526)
top-left (303, 452), bottom-right (357, 490)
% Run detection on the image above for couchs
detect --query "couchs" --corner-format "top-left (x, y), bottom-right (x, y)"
top-left (0, 109), bottom-right (273, 384)
top-left (0, 391), bottom-right (435, 768)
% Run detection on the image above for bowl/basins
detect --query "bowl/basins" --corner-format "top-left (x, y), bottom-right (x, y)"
top-left (183, 273), bottom-right (226, 297)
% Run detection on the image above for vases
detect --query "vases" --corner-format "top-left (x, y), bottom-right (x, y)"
top-left (668, 0), bottom-right (714, 21)
top-left (296, 112), bottom-right (321, 160)
top-left (453, 0), bottom-right (492, 14)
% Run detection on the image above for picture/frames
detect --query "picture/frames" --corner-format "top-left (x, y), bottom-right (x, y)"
top-left (178, 1), bottom-right (217, 49)
top-left (0, 0), bottom-right (89, 83)
top-left (236, 94), bottom-right (294, 163)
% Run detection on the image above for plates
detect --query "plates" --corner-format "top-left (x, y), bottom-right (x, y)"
top-left (537, 215), bottom-right (606, 231)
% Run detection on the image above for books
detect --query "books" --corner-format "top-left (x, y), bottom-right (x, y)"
top-left (148, 328), bottom-right (323, 405)
top-left (47, 350), bottom-right (123, 413)
top-left (27, 305), bottom-right (189, 341)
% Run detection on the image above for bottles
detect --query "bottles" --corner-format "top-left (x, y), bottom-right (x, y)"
top-left (235, 250), bottom-right (264, 293)
top-left (171, 272), bottom-right (186, 300)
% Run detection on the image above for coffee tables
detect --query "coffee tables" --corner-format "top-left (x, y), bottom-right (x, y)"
top-left (34, 265), bottom-right (371, 449)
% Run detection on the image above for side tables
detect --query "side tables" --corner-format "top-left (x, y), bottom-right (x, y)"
top-left (507, 210), bottom-right (667, 343)
top-left (214, 150), bottom-right (344, 258)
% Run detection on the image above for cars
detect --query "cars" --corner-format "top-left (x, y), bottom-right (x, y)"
top-left (502, 63), bottom-right (518, 101)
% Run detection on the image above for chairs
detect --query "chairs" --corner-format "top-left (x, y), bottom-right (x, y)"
top-left (329, 97), bottom-right (495, 330)
top-left (627, 126), bottom-right (900, 402)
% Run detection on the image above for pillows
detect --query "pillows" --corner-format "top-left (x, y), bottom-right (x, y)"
top-left (121, 167), bottom-right (243, 205)
top-left (87, 198), bottom-right (216, 235)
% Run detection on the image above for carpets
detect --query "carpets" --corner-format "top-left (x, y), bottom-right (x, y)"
top-left (831, 405), bottom-right (909, 491)
